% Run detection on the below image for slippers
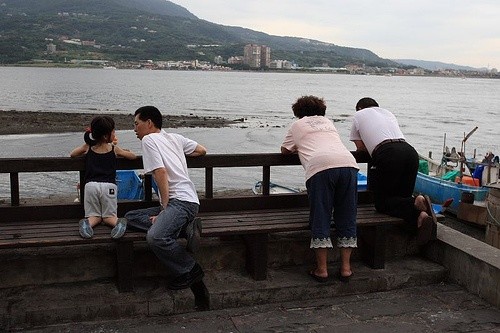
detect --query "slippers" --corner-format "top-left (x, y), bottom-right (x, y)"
top-left (339, 271), bottom-right (353, 280)
top-left (308, 268), bottom-right (328, 282)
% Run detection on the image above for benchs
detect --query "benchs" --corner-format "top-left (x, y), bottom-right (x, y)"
top-left (0, 202), bottom-right (404, 291)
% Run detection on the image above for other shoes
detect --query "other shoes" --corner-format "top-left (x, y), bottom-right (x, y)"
top-left (78, 219), bottom-right (94, 238)
top-left (173, 264), bottom-right (205, 290)
top-left (111, 218), bottom-right (128, 238)
top-left (185, 218), bottom-right (202, 252)
top-left (414, 216), bottom-right (433, 253)
top-left (424, 194), bottom-right (437, 239)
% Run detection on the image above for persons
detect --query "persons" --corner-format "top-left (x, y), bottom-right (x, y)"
top-left (349, 97), bottom-right (437, 247)
top-left (124, 105), bottom-right (206, 290)
top-left (70, 117), bottom-right (137, 241)
top-left (281, 96), bottom-right (359, 283)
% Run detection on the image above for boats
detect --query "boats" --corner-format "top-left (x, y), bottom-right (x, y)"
top-left (356, 171), bottom-right (454, 215)
top-left (413, 151), bottom-right (489, 207)
top-left (430, 127), bottom-right (500, 190)
